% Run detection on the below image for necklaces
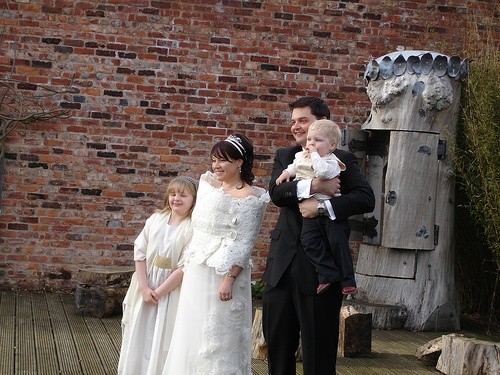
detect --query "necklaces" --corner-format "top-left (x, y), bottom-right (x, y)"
top-left (220, 180), bottom-right (243, 191)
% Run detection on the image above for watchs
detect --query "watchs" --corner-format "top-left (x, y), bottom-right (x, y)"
top-left (316, 200), bottom-right (325, 215)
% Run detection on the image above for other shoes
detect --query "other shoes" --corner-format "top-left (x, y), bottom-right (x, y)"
top-left (342, 286), bottom-right (356, 294)
top-left (316, 283), bottom-right (331, 295)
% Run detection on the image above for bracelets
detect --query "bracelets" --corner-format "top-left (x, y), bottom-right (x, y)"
top-left (225, 272), bottom-right (237, 280)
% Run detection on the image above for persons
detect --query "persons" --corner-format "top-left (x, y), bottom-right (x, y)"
top-left (260, 96), bottom-right (376, 375)
top-left (275, 119), bottom-right (358, 294)
top-left (160, 134), bottom-right (271, 375)
top-left (117, 177), bottom-right (199, 375)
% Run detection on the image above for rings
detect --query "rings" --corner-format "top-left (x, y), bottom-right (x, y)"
top-left (301, 213), bottom-right (305, 218)
top-left (299, 208), bottom-right (302, 212)
